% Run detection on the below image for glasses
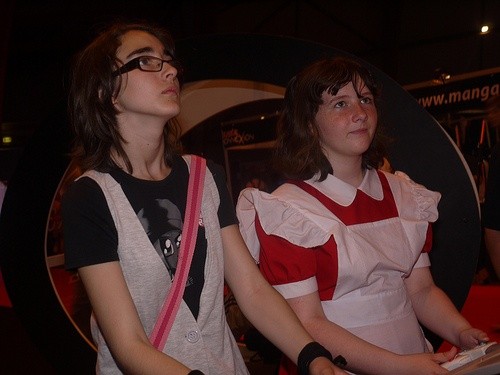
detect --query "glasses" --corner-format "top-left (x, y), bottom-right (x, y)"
top-left (112, 55), bottom-right (185, 77)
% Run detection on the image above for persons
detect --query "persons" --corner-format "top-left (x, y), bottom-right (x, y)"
top-left (231, 170), bottom-right (271, 206)
top-left (479, 93), bottom-right (500, 287)
top-left (60, 25), bottom-right (356, 375)
top-left (0, 99), bottom-right (60, 331)
top-left (233, 55), bottom-right (489, 375)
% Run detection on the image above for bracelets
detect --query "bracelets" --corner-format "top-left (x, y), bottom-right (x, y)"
top-left (297, 342), bottom-right (348, 375)
top-left (188, 369), bottom-right (204, 375)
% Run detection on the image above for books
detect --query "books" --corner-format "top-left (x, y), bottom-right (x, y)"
top-left (441, 342), bottom-right (495, 372)
